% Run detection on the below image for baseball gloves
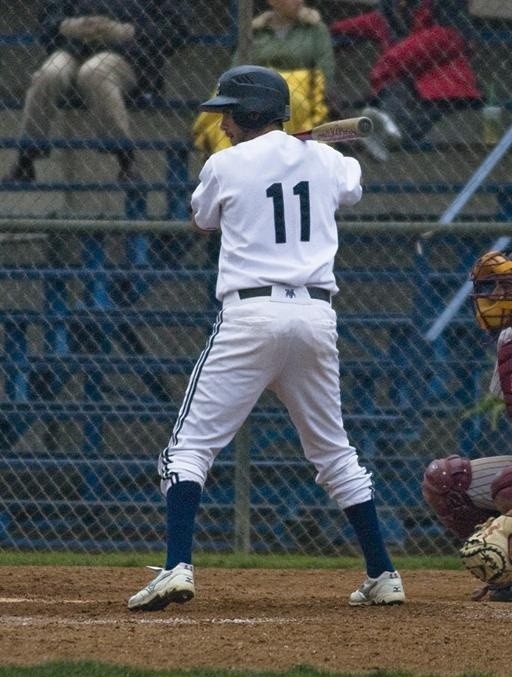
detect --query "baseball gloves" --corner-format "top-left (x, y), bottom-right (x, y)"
top-left (459, 509), bottom-right (512, 586)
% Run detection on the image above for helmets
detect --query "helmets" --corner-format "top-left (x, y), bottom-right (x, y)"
top-left (195, 64), bottom-right (292, 132)
top-left (470, 247), bottom-right (512, 332)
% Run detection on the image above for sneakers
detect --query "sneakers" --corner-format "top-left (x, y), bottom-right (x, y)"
top-left (346, 568), bottom-right (407, 607)
top-left (347, 107), bottom-right (406, 166)
top-left (114, 147), bottom-right (142, 185)
top-left (5, 152), bottom-right (40, 186)
top-left (124, 561), bottom-right (198, 611)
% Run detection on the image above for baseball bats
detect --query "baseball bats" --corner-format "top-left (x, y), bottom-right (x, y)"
top-left (290, 117), bottom-right (374, 142)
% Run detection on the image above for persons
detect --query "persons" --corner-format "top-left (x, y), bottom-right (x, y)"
top-left (126, 64), bottom-right (408, 611)
top-left (213, 0), bottom-right (337, 82)
top-left (2, 0), bottom-right (182, 192)
top-left (418, 247), bottom-right (511, 603)
top-left (328, 0), bottom-right (485, 154)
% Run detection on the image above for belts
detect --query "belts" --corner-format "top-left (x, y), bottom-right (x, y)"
top-left (237, 284), bottom-right (334, 304)
